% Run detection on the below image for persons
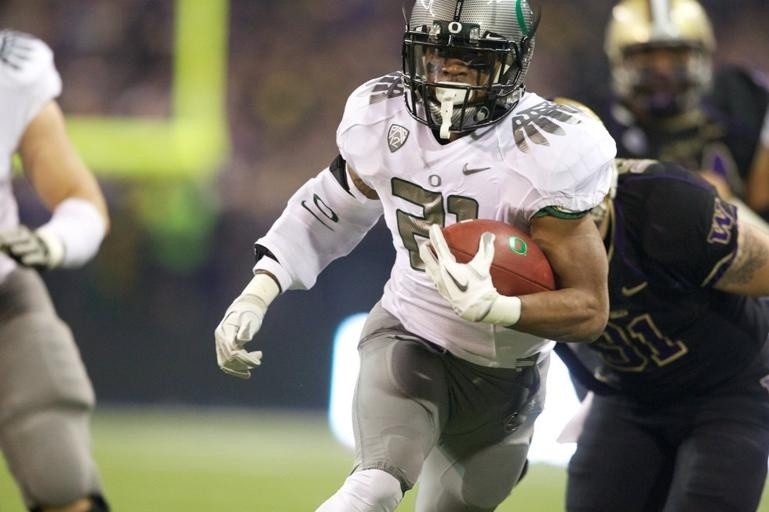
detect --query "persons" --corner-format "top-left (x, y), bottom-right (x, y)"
top-left (0, 30), bottom-right (117, 509)
top-left (589, 0), bottom-right (767, 208)
top-left (214, 0), bottom-right (618, 511)
top-left (532, 96), bottom-right (767, 512)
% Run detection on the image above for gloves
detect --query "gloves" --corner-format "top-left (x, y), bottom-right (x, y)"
top-left (419, 223), bottom-right (521, 328)
top-left (214, 273), bottom-right (281, 380)
top-left (0, 223), bottom-right (49, 268)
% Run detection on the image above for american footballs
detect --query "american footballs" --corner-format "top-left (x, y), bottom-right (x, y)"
top-left (428, 219), bottom-right (556, 297)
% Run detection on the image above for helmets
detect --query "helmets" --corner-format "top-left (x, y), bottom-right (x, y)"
top-left (603, 0), bottom-right (717, 121)
top-left (400, 0), bottom-right (542, 140)
top-left (548, 96), bottom-right (611, 240)
